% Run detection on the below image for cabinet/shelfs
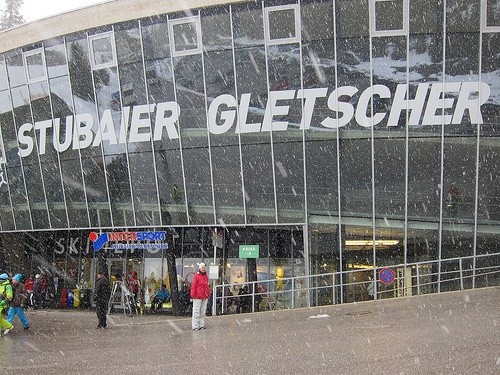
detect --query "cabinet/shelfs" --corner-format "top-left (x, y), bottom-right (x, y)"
top-left (107, 280), bottom-right (131, 318)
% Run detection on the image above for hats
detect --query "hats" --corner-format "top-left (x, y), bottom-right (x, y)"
top-left (12, 273), bottom-right (22, 281)
top-left (0, 273), bottom-right (8, 282)
top-left (199, 262), bottom-right (207, 270)
top-left (96, 269), bottom-right (104, 274)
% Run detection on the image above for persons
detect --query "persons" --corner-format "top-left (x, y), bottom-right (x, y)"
top-left (190, 262), bottom-right (212, 331)
top-left (6, 273), bottom-right (30, 331)
top-left (0, 271), bottom-right (14, 337)
top-left (91, 268), bottom-right (111, 329)
top-left (109, 271), bottom-right (264, 315)
top-left (24, 268), bottom-right (92, 315)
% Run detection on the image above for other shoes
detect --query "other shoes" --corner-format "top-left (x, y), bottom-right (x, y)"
top-left (3, 326), bottom-right (14, 335)
top-left (194, 326), bottom-right (206, 331)
top-left (96, 324), bottom-right (106, 329)
top-left (22, 326), bottom-right (30, 331)
